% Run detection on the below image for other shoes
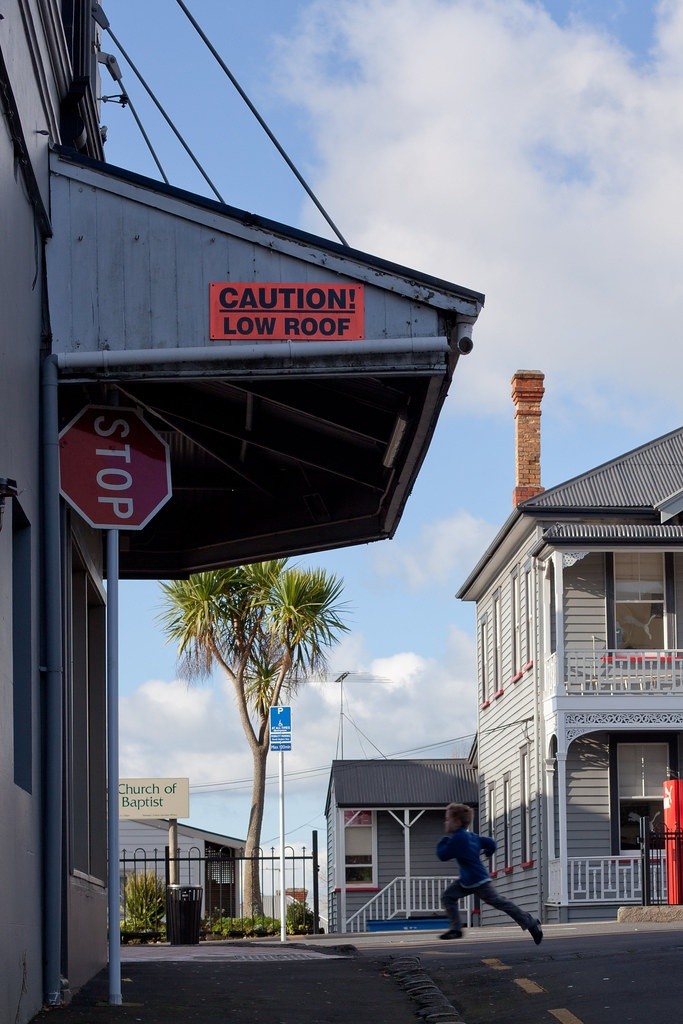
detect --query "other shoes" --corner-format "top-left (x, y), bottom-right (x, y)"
top-left (440, 930), bottom-right (462, 940)
top-left (528, 920), bottom-right (543, 944)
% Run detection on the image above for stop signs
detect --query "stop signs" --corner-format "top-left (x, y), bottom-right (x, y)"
top-left (58, 405), bottom-right (173, 530)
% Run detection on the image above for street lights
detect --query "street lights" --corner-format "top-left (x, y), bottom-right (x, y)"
top-left (333, 671), bottom-right (349, 760)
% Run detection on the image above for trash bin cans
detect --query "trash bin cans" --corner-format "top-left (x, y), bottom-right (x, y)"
top-left (166, 882), bottom-right (203, 945)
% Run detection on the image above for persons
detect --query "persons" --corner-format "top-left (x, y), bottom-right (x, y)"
top-left (434, 803), bottom-right (543, 945)
